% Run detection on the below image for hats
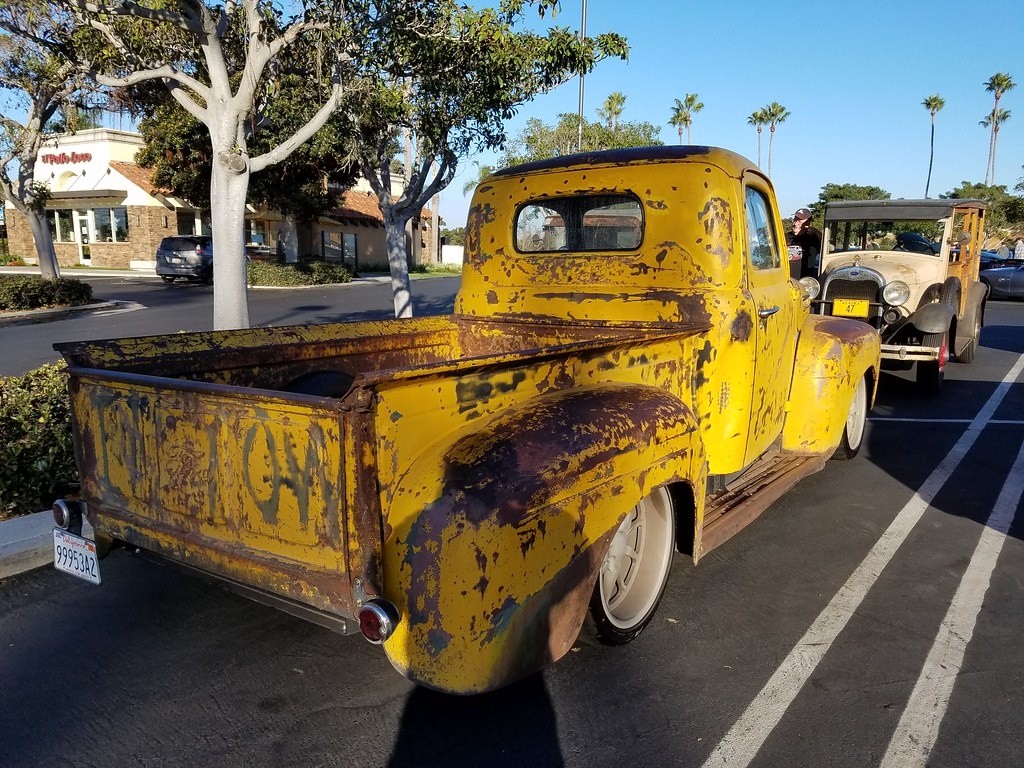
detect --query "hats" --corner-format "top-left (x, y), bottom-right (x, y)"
top-left (790, 209), bottom-right (812, 221)
top-left (1014, 237), bottom-right (1022, 242)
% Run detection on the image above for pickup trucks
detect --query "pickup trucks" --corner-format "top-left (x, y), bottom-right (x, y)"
top-left (52, 144), bottom-right (881, 698)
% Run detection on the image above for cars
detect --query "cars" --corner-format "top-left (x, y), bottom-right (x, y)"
top-left (978, 258), bottom-right (1024, 302)
top-left (932, 243), bottom-right (1006, 266)
top-left (245, 244), bottom-right (278, 261)
top-left (798, 197), bottom-right (986, 390)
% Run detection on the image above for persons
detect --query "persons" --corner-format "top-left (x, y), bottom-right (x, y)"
top-left (784, 208), bottom-right (823, 313)
top-left (996, 242), bottom-right (1009, 259)
top-left (1013, 237), bottom-right (1024, 259)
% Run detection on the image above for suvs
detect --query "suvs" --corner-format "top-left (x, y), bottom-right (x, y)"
top-left (156, 234), bottom-right (213, 284)
